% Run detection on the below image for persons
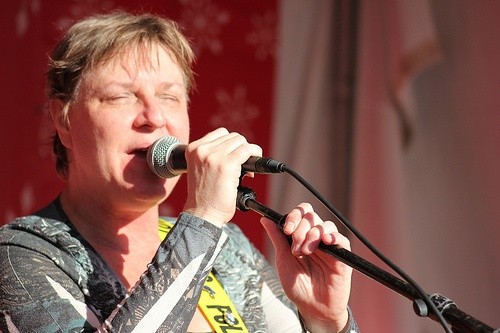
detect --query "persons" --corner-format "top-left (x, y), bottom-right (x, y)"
top-left (0, 11), bottom-right (362, 333)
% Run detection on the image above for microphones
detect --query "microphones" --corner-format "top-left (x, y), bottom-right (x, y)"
top-left (147, 137), bottom-right (283, 180)
